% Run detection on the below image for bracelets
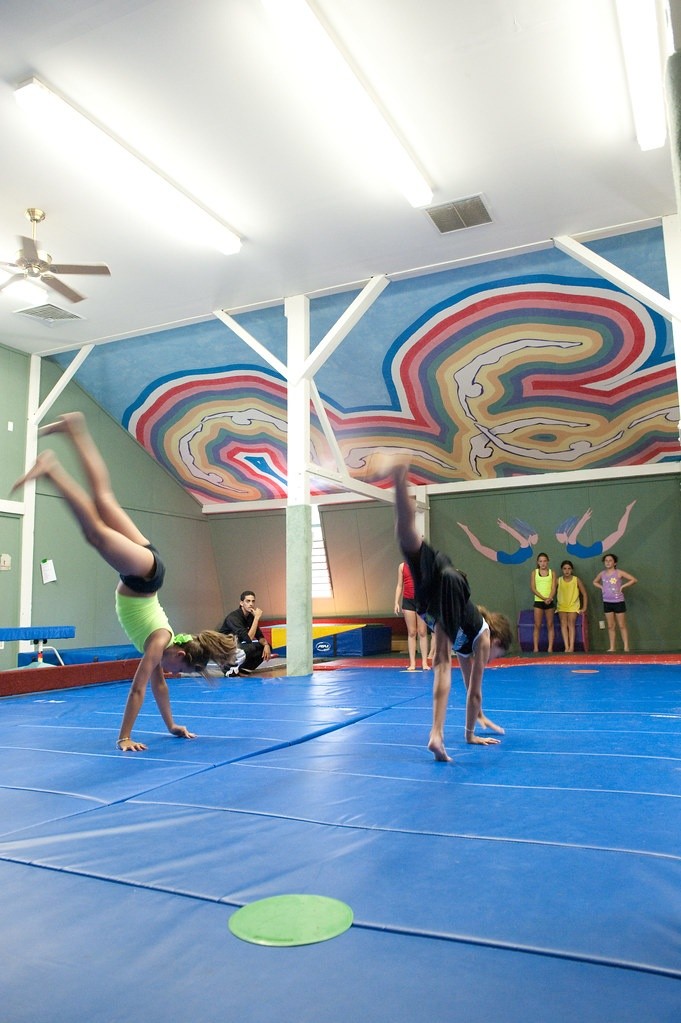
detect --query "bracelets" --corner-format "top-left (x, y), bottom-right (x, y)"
top-left (465, 727), bottom-right (475, 732)
top-left (116, 738), bottom-right (129, 744)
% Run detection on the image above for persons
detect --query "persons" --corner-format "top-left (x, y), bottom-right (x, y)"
top-left (395, 560), bottom-right (431, 670)
top-left (593, 554), bottom-right (637, 652)
top-left (531, 553), bottom-right (556, 652)
top-left (9, 411), bottom-right (236, 751)
top-left (555, 560), bottom-right (587, 652)
top-left (372, 454), bottom-right (510, 761)
top-left (219, 591), bottom-right (270, 677)
